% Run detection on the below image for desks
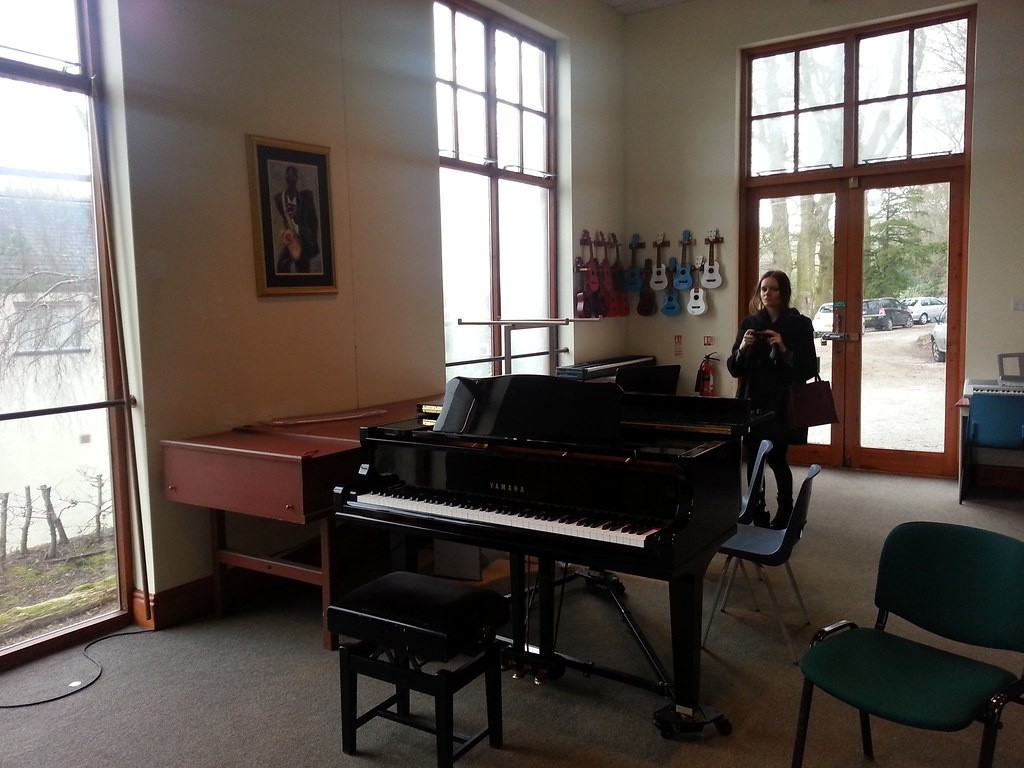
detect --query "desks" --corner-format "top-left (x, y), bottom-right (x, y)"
top-left (955, 396), bottom-right (970, 505)
top-left (161, 392), bottom-right (445, 651)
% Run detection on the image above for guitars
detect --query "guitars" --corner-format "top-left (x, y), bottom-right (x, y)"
top-left (673, 230), bottom-right (693, 291)
top-left (686, 255), bottom-right (709, 316)
top-left (660, 257), bottom-right (682, 317)
top-left (582, 230), bottom-right (599, 294)
top-left (700, 227), bottom-right (722, 289)
top-left (626, 233), bottom-right (642, 294)
top-left (597, 230), bottom-right (613, 294)
top-left (575, 257), bottom-right (591, 318)
top-left (650, 232), bottom-right (669, 292)
top-left (610, 232), bottom-right (626, 293)
top-left (637, 259), bottom-right (658, 316)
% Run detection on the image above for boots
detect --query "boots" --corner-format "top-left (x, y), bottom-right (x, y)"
top-left (739, 487), bottom-right (765, 527)
top-left (769, 492), bottom-right (793, 530)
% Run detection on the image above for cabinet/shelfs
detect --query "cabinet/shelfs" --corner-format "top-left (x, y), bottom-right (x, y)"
top-left (244, 133), bottom-right (339, 298)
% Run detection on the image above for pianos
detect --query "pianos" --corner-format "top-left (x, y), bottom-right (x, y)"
top-left (327, 373), bottom-right (749, 738)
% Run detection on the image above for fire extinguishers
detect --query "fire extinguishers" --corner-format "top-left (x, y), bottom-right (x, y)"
top-left (694, 351), bottom-right (721, 396)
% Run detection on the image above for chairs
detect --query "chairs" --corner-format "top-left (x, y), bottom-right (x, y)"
top-left (791, 522), bottom-right (1024, 768)
top-left (615, 364), bottom-right (681, 395)
top-left (702, 464), bottom-right (822, 665)
top-left (720, 439), bottom-right (773, 612)
top-left (958, 392), bottom-right (1024, 501)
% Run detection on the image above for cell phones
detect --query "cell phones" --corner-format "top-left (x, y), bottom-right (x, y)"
top-left (751, 332), bottom-right (773, 341)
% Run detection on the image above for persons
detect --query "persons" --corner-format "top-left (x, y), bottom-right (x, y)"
top-left (270, 164), bottom-right (319, 273)
top-left (727, 270), bottom-right (817, 531)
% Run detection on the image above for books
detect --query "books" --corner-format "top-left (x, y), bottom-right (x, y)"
top-left (416, 399), bottom-right (443, 413)
top-left (416, 415), bottom-right (437, 426)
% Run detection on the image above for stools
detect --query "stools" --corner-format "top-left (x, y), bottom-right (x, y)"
top-left (326, 572), bottom-right (508, 768)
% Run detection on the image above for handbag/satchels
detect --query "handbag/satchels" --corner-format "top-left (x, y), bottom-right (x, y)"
top-left (788, 359), bottom-right (840, 430)
